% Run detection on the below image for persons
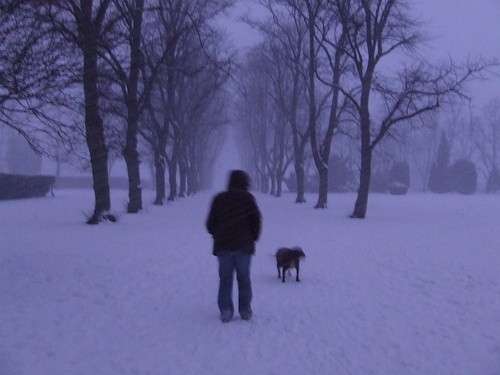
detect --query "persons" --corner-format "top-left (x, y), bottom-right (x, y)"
top-left (206, 169), bottom-right (262, 322)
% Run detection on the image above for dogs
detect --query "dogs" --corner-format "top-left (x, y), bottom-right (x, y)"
top-left (276, 246), bottom-right (306, 283)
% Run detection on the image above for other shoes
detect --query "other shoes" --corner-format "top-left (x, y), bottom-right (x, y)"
top-left (241, 311), bottom-right (252, 320)
top-left (221, 312), bottom-right (234, 322)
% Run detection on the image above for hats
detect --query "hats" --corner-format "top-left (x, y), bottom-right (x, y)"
top-left (228, 170), bottom-right (251, 189)
